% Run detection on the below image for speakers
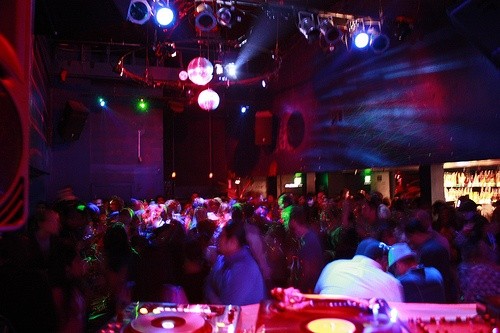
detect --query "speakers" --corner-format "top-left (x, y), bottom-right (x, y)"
top-left (59, 100), bottom-right (88, 142)
top-left (247, 109), bottom-right (273, 146)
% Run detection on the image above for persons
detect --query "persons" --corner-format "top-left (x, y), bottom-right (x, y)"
top-left (38, 185), bottom-right (500, 333)
top-left (313, 238), bottom-right (403, 302)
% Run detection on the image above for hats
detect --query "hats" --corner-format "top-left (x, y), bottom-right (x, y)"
top-left (388, 242), bottom-right (416, 268)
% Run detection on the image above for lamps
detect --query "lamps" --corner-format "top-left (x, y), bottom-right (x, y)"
top-left (127, 0), bottom-right (411, 52)
